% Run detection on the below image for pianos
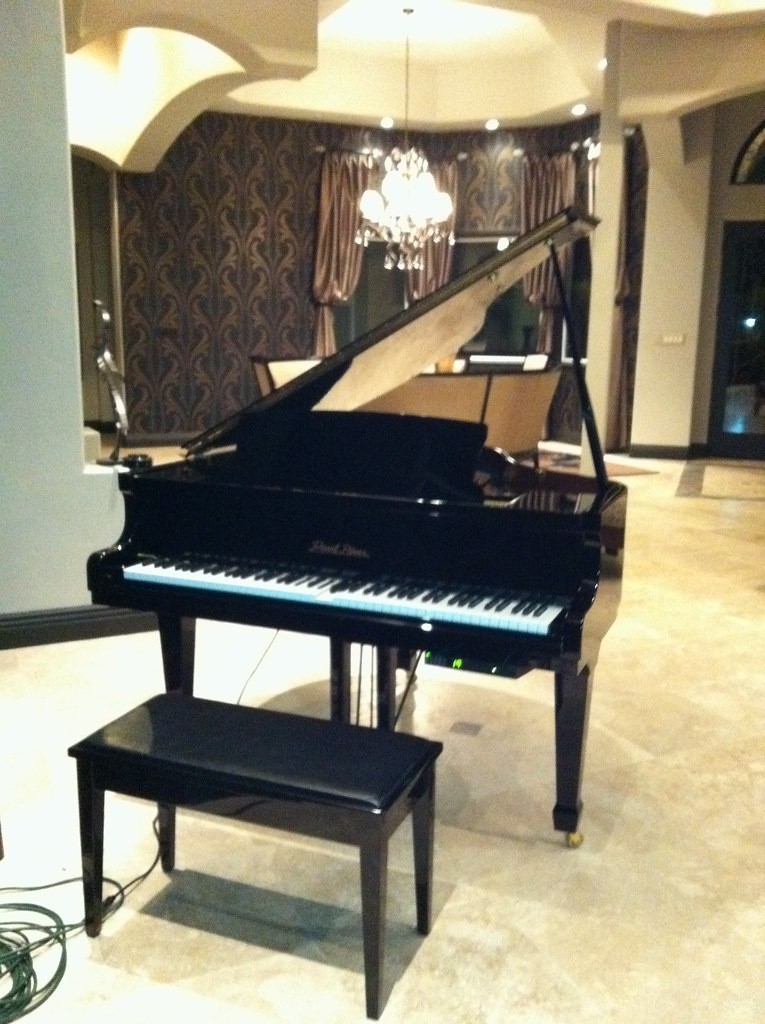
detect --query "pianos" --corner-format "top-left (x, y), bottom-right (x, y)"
top-left (83, 206), bottom-right (630, 850)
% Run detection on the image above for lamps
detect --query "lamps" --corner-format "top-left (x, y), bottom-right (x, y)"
top-left (354, 8), bottom-right (459, 273)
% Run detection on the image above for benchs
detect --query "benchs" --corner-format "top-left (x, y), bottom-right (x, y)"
top-left (67, 694), bottom-right (439, 1018)
